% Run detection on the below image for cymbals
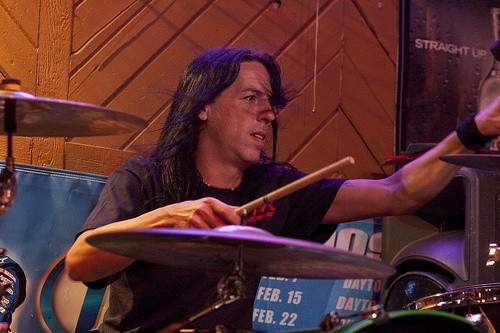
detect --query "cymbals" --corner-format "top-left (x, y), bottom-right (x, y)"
top-left (439, 154), bottom-right (500, 172)
top-left (85, 225), bottom-right (397, 279)
top-left (0, 90), bottom-right (149, 137)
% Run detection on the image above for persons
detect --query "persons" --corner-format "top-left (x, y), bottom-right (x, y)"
top-left (65, 49), bottom-right (500, 333)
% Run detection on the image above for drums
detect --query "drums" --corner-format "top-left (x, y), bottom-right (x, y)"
top-left (319, 283), bottom-right (500, 333)
